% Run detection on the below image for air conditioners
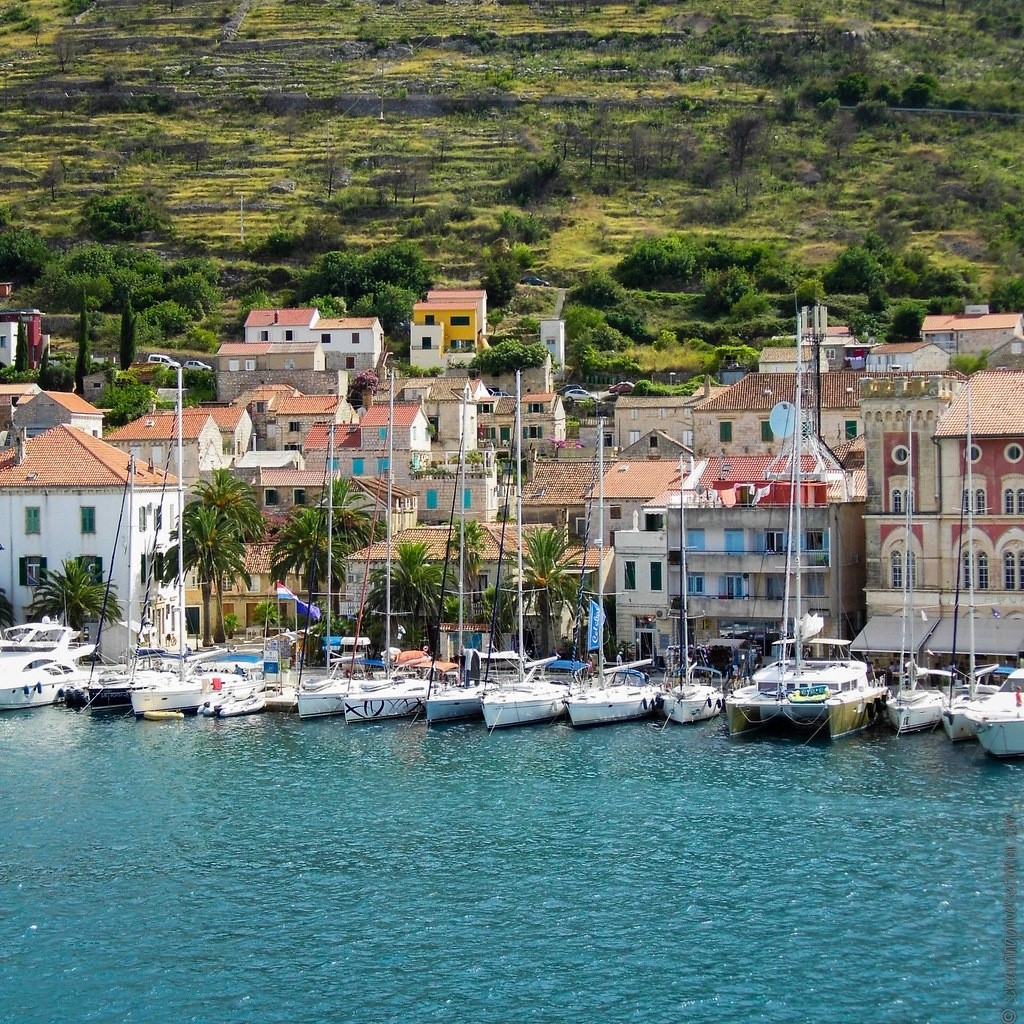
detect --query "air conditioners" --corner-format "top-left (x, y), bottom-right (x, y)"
top-left (853, 611), bottom-right (863, 630)
top-left (654, 609), bottom-right (668, 620)
top-left (854, 554), bottom-right (862, 564)
top-left (742, 573), bottom-right (750, 580)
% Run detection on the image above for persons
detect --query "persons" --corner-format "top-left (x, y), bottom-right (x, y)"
top-left (865, 659), bottom-right (1010, 686)
top-left (580, 651), bottom-right (623, 673)
top-left (689, 643), bottom-right (733, 679)
top-left (1016, 686), bottom-right (1023, 718)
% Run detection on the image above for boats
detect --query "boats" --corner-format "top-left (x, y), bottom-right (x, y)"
top-left (0, 586), bottom-right (102, 710)
top-left (198, 692), bottom-right (267, 718)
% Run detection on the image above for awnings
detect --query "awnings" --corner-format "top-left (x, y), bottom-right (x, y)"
top-left (850, 616), bottom-right (1024, 656)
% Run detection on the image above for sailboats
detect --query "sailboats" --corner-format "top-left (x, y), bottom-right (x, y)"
top-left (885, 415), bottom-right (951, 734)
top-left (658, 451), bottom-right (722, 725)
top-left (563, 417), bottom-right (660, 727)
top-left (943, 380), bottom-right (1024, 759)
top-left (425, 394), bottom-right (483, 724)
top-left (294, 422), bottom-right (344, 721)
top-left (341, 371), bottom-right (430, 725)
top-left (725, 292), bottom-right (890, 742)
top-left (131, 366), bottom-right (266, 720)
top-left (86, 454), bottom-right (153, 711)
top-left (480, 370), bottom-right (571, 729)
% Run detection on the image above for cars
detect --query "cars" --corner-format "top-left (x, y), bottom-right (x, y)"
top-left (609, 382), bottom-right (635, 393)
top-left (556, 384), bottom-right (581, 396)
top-left (148, 354), bottom-right (182, 367)
top-left (564, 389), bottom-right (598, 401)
top-left (184, 360), bottom-right (212, 371)
top-left (521, 275), bottom-right (551, 286)
top-left (491, 392), bottom-right (512, 397)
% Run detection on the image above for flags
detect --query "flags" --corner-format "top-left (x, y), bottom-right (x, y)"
top-left (589, 598), bottom-right (605, 651)
top-left (277, 583), bottom-right (295, 600)
top-left (296, 598), bottom-right (320, 619)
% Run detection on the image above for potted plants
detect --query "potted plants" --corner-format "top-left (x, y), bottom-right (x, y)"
top-left (252, 601), bottom-right (283, 637)
top-left (224, 613), bottom-right (243, 639)
